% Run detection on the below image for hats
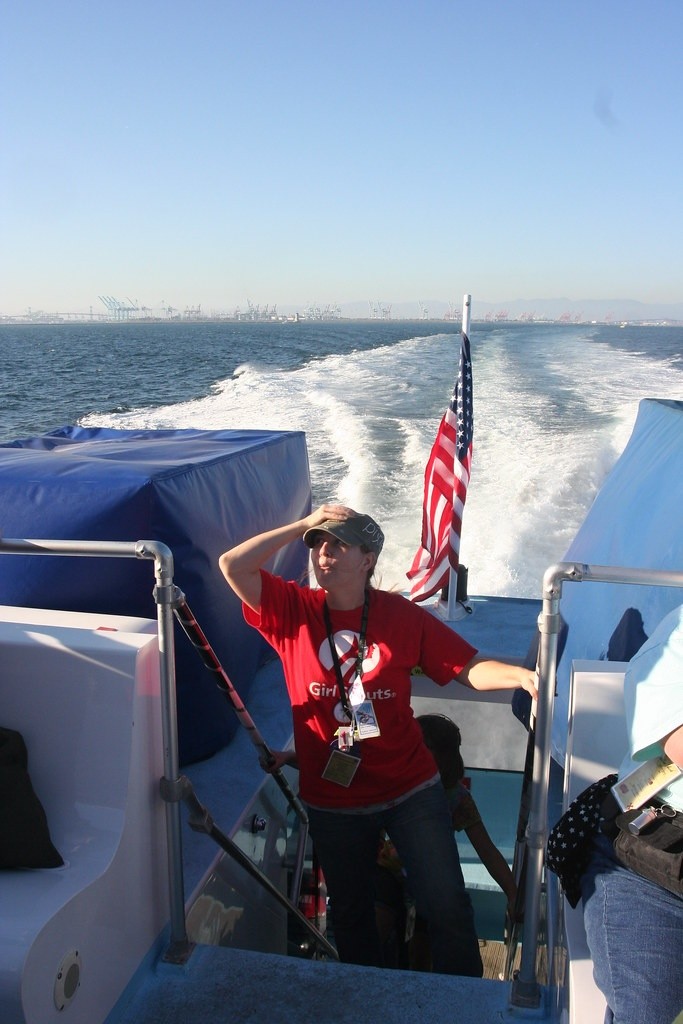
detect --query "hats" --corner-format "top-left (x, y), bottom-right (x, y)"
top-left (302, 513), bottom-right (385, 560)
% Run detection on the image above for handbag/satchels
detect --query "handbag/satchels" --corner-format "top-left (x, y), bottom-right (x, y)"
top-left (613, 799), bottom-right (683, 900)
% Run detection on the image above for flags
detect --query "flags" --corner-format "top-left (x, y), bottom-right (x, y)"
top-left (404, 332), bottom-right (474, 604)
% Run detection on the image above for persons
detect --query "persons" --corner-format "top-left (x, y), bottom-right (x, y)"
top-left (219, 502), bottom-right (539, 982)
top-left (542, 606), bottom-right (683, 1024)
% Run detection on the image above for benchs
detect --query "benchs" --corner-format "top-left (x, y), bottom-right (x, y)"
top-left (0, 619), bottom-right (170, 1023)
top-left (559, 658), bottom-right (629, 1023)
